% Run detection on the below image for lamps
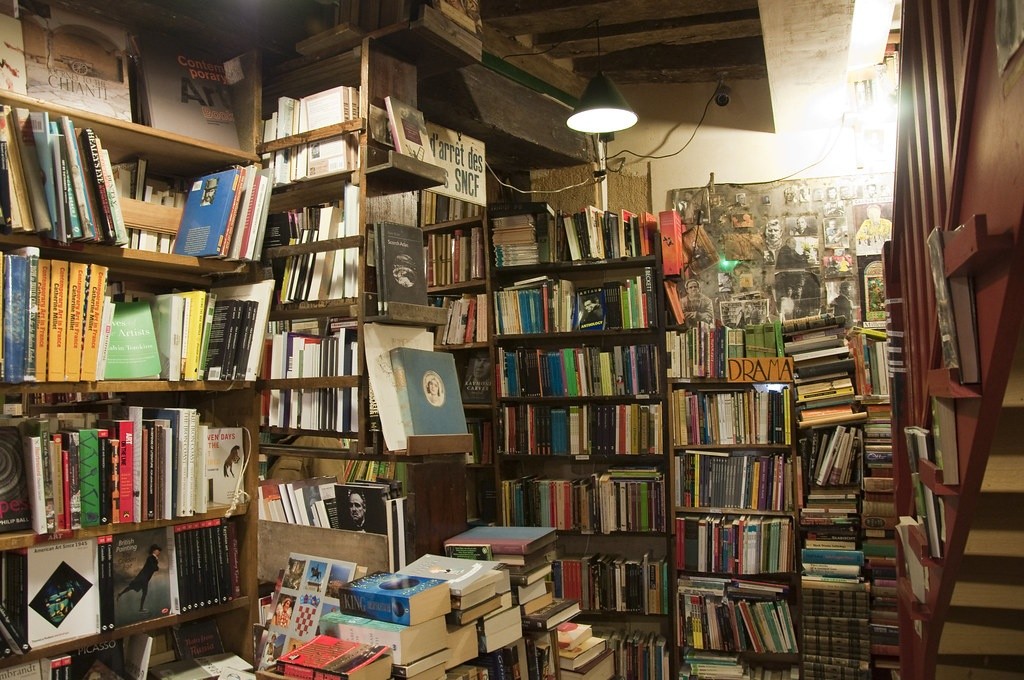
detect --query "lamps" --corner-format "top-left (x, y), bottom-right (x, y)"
top-left (566, 20), bottom-right (640, 134)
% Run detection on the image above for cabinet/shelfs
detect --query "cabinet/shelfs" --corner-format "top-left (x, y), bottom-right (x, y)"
top-left (423, 208), bottom-right (503, 526)
top-left (492, 233), bottom-right (679, 680)
top-left (257, 36), bottom-right (473, 597)
top-left (667, 378), bottom-right (804, 680)
top-left (0, 0), bottom-right (256, 680)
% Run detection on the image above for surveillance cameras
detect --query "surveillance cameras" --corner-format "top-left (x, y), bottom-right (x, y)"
top-left (715, 85), bottom-right (730, 106)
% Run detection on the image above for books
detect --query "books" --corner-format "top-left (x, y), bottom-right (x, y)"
top-left (0, 0), bottom-right (975, 680)
